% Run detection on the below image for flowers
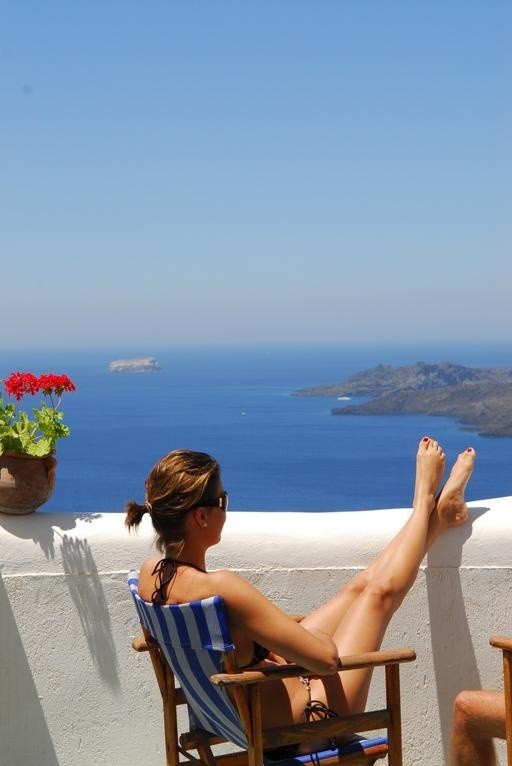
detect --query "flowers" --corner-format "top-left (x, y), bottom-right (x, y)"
top-left (0, 368), bottom-right (73, 461)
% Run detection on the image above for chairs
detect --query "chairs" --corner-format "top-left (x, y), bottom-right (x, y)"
top-left (126, 569), bottom-right (421, 765)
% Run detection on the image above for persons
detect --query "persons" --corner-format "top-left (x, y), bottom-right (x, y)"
top-left (450, 689), bottom-right (506, 766)
top-left (124, 436), bottom-right (477, 761)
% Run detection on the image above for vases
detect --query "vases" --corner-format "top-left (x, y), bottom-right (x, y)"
top-left (0, 451), bottom-right (55, 517)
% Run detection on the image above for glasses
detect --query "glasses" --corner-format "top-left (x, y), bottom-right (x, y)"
top-left (199, 491), bottom-right (229, 513)
